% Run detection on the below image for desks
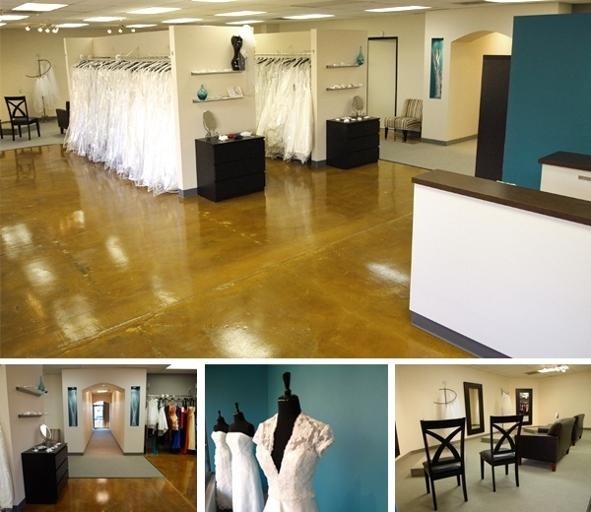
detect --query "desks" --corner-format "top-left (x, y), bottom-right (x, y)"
top-left (326, 116), bottom-right (380, 169)
top-left (195, 132), bottom-right (265, 202)
top-left (22, 441), bottom-right (69, 504)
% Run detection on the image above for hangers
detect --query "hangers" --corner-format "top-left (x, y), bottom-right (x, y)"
top-left (147, 394), bottom-right (194, 407)
top-left (256, 56), bottom-right (310, 68)
top-left (71, 56), bottom-right (172, 78)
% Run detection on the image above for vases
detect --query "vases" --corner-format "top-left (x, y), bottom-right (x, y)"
top-left (356, 46), bottom-right (365, 64)
top-left (197, 84), bottom-right (207, 100)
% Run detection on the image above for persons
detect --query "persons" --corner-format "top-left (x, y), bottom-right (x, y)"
top-left (226, 411), bottom-right (265, 512)
top-left (252, 394), bottom-right (336, 512)
top-left (211, 417), bottom-right (230, 511)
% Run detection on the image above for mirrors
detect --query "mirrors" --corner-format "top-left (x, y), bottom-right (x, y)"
top-left (464, 382), bottom-right (483, 436)
top-left (39, 423), bottom-right (53, 445)
top-left (515, 388), bottom-right (532, 425)
top-left (203, 110), bottom-right (219, 138)
top-left (352, 95), bottom-right (364, 118)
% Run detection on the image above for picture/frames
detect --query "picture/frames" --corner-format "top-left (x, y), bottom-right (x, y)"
top-left (427, 35), bottom-right (445, 101)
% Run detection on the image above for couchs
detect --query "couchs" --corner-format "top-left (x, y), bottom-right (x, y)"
top-left (383, 98), bottom-right (424, 143)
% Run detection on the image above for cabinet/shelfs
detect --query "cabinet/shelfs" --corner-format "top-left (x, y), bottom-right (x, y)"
top-left (16, 386), bottom-right (43, 418)
top-left (326, 65), bottom-right (363, 90)
top-left (191, 70), bottom-right (244, 102)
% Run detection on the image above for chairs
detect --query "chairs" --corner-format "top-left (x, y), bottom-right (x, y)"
top-left (480, 413), bottom-right (525, 492)
top-left (515, 414), bottom-right (585, 472)
top-left (56, 101), bottom-right (70, 134)
top-left (4, 96), bottom-right (42, 141)
top-left (419, 416), bottom-right (468, 510)
